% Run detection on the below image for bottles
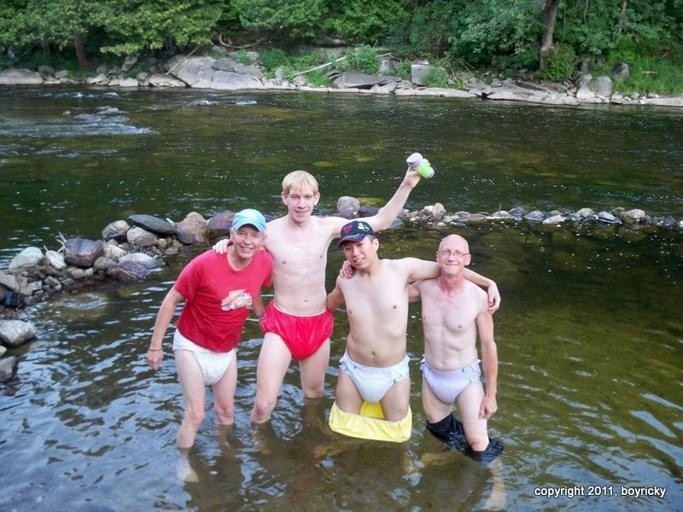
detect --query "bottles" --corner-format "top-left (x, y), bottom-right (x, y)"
top-left (406, 152), bottom-right (435, 182)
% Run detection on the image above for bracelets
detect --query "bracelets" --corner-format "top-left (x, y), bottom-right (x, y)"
top-left (149, 348), bottom-right (163, 352)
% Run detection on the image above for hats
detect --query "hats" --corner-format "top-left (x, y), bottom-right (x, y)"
top-left (233, 209), bottom-right (266, 232)
top-left (337, 221), bottom-right (377, 250)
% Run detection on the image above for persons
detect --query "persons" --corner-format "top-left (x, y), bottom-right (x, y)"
top-left (146, 209), bottom-right (274, 482)
top-left (338, 234), bottom-right (498, 451)
top-left (210, 157), bottom-right (432, 425)
top-left (325, 219), bottom-right (502, 420)
